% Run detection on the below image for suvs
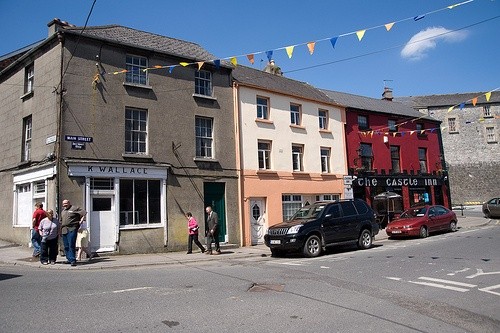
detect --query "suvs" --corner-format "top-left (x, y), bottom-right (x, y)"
top-left (264, 198), bottom-right (379, 258)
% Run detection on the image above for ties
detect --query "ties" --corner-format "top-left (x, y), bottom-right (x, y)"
top-left (208, 213), bottom-right (211, 220)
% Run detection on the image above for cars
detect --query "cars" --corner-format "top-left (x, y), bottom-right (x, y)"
top-left (481, 197), bottom-right (500, 219)
top-left (386, 204), bottom-right (458, 239)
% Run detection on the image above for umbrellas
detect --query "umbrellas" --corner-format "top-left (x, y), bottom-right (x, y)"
top-left (369, 189), bottom-right (402, 223)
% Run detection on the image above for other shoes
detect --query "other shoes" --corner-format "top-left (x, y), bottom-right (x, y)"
top-left (71, 261), bottom-right (76, 266)
top-left (205, 251), bottom-right (212, 255)
top-left (50, 260), bottom-right (56, 264)
top-left (42, 262), bottom-right (48, 265)
top-left (215, 251), bottom-right (221, 255)
top-left (76, 258), bottom-right (81, 261)
top-left (202, 248), bottom-right (205, 253)
top-left (63, 261), bottom-right (71, 264)
top-left (187, 252), bottom-right (191, 254)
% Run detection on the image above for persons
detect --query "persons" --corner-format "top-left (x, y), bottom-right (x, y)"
top-left (31, 202), bottom-right (47, 256)
top-left (185, 212), bottom-right (206, 254)
top-left (76, 222), bottom-right (94, 262)
top-left (38, 209), bottom-right (60, 265)
top-left (59, 200), bottom-right (87, 267)
top-left (205, 206), bottom-right (221, 255)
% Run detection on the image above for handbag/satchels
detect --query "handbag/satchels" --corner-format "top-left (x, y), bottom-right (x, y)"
top-left (191, 226), bottom-right (199, 232)
